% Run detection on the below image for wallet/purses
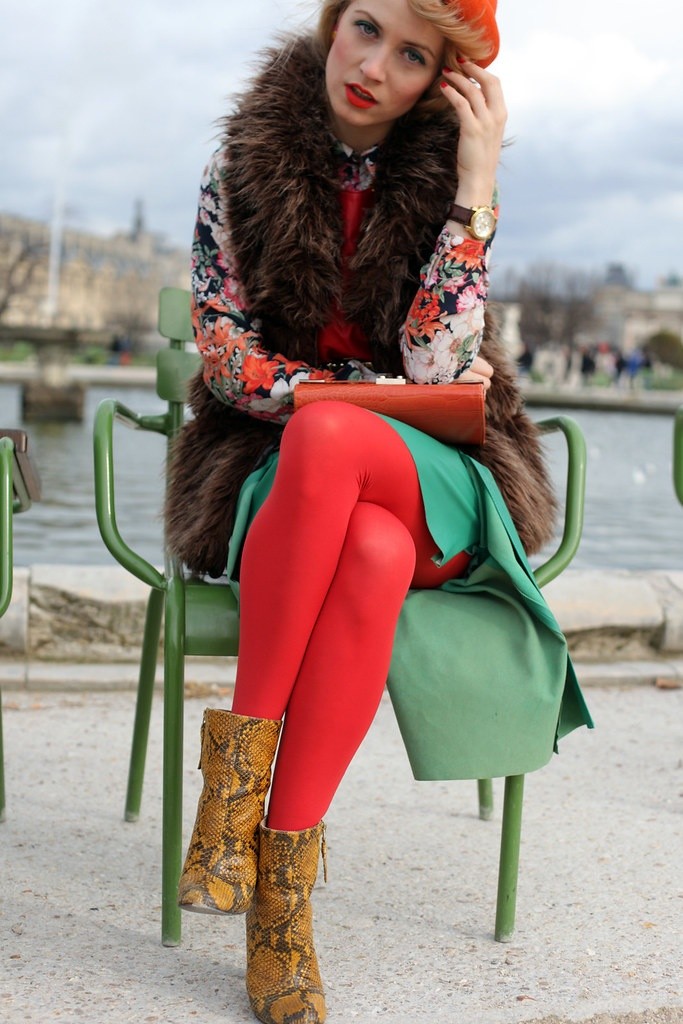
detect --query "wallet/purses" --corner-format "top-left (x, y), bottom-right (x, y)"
top-left (293, 380), bottom-right (488, 447)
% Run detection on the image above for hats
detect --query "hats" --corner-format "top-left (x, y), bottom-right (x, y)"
top-left (443, 0), bottom-right (501, 89)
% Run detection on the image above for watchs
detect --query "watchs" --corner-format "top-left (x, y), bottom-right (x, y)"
top-left (444, 201), bottom-right (497, 240)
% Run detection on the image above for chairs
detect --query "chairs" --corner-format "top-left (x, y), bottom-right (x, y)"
top-left (92, 285), bottom-right (584, 948)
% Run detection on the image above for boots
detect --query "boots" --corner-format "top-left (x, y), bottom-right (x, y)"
top-left (177, 707), bottom-right (282, 916)
top-left (245, 817), bottom-right (328, 1024)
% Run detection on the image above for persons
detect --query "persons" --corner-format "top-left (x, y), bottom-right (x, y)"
top-left (581, 347), bottom-right (595, 385)
top-left (162, 2), bottom-right (504, 1023)
top-left (515, 341), bottom-right (532, 374)
top-left (615, 348), bottom-right (653, 387)
